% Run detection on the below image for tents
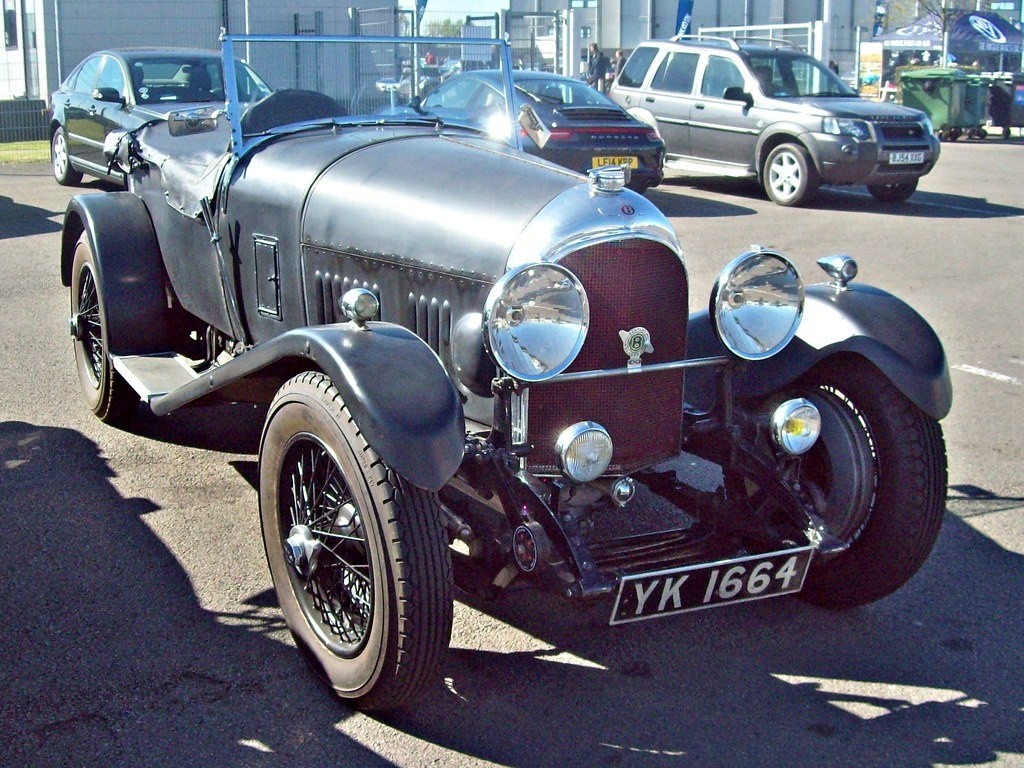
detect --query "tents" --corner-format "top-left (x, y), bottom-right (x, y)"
top-left (872, 9), bottom-right (1024, 99)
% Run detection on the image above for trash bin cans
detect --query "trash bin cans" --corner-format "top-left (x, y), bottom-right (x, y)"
top-left (900, 67), bottom-right (970, 141)
top-left (992, 71), bottom-right (1024, 142)
top-left (968, 74), bottom-right (991, 140)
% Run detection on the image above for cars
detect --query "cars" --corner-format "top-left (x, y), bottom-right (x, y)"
top-left (370, 68), bottom-right (667, 195)
top-left (59, 29), bottom-right (954, 706)
top-left (45, 45), bottom-right (275, 190)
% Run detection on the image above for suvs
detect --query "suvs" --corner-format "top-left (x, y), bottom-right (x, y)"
top-left (606, 33), bottom-right (944, 208)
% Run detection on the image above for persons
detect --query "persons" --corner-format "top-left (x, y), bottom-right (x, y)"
top-left (591, 51), bottom-right (607, 94)
top-left (425, 52), bottom-right (436, 65)
top-left (615, 50), bottom-right (626, 79)
top-left (590, 43), bottom-right (599, 74)
top-left (830, 51), bottom-right (984, 93)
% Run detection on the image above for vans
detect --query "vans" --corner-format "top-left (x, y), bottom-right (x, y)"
top-left (371, 63), bottom-right (443, 107)
